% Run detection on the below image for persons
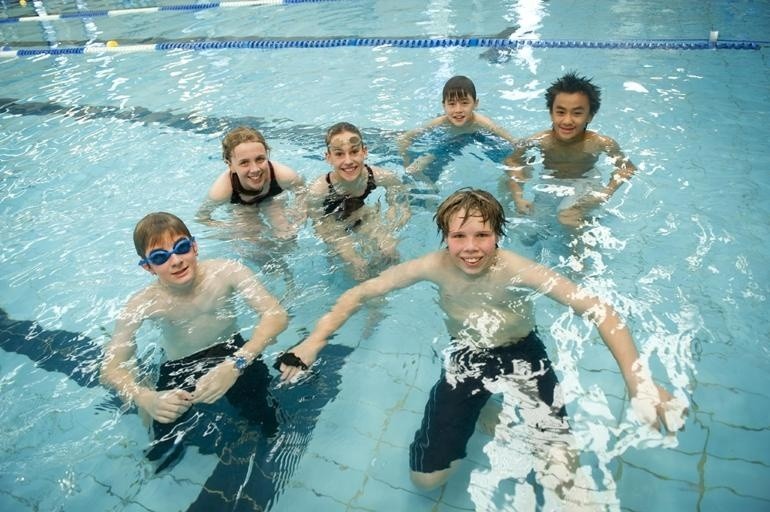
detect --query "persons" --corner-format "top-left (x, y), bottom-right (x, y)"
top-left (298, 123), bottom-right (410, 280)
top-left (196, 125), bottom-right (308, 276)
top-left (506, 73), bottom-right (636, 246)
top-left (280, 187), bottom-right (685, 491)
top-left (397, 75), bottom-right (518, 193)
top-left (100, 213), bottom-right (289, 462)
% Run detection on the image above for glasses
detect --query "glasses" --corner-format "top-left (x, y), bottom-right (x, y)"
top-left (273, 353), bottom-right (319, 386)
top-left (327, 135), bottom-right (364, 149)
top-left (139, 235), bottom-right (195, 268)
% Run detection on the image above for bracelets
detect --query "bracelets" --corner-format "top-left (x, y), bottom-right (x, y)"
top-left (226, 354), bottom-right (248, 372)
